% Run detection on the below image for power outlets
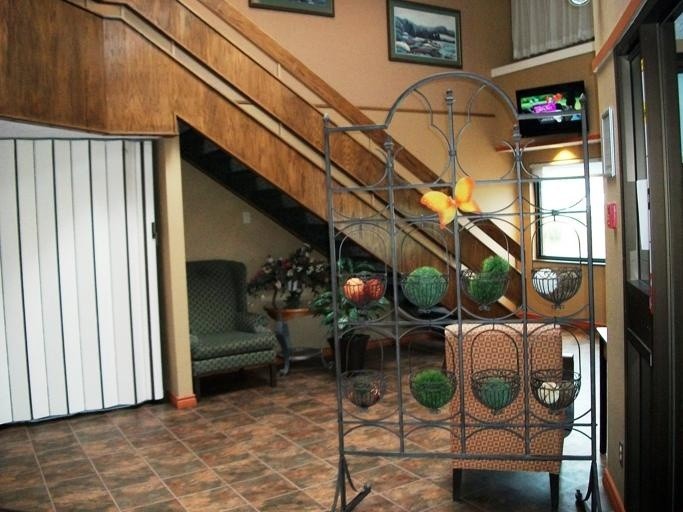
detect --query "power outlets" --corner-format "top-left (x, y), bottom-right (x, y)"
top-left (618, 441), bottom-right (624, 469)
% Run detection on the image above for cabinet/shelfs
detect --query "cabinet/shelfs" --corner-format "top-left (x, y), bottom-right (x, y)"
top-left (615, 1), bottom-right (683, 509)
top-left (323, 72), bottom-right (601, 512)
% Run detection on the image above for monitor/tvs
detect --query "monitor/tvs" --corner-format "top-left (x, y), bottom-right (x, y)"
top-left (515, 80), bottom-right (588, 138)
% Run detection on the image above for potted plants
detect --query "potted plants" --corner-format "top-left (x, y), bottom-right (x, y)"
top-left (308, 258), bottom-right (391, 378)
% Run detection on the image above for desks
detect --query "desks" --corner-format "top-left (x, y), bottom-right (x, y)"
top-left (264, 302), bottom-right (326, 375)
top-left (595, 326), bottom-right (607, 454)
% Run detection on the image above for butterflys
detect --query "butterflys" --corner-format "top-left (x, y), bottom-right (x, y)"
top-left (420, 176), bottom-right (481, 230)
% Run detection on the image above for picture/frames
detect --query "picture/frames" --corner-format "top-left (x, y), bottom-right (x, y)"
top-left (249, 0), bottom-right (335, 17)
top-left (600, 106), bottom-right (616, 178)
top-left (387, 1), bottom-right (463, 68)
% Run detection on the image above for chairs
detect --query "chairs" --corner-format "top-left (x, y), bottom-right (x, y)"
top-left (187, 261), bottom-right (278, 401)
top-left (445, 324), bottom-right (562, 511)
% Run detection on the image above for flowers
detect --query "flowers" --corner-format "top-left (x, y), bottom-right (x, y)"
top-left (247, 242), bottom-right (331, 302)
